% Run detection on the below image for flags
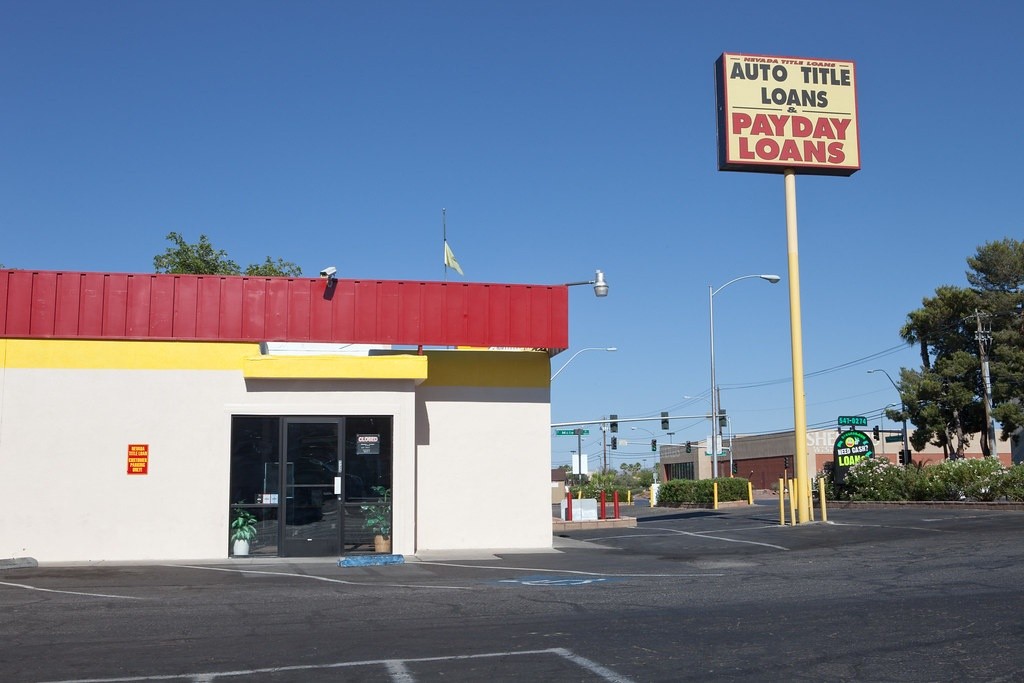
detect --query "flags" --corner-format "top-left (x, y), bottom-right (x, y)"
top-left (443, 243), bottom-right (464, 276)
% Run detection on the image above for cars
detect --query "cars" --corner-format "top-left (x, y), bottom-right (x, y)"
top-left (240, 454), bottom-right (362, 501)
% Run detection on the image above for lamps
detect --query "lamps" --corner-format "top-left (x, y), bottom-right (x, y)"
top-left (565, 270), bottom-right (609, 296)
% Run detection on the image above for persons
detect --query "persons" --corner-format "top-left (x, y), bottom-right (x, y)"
top-left (951, 448), bottom-right (965, 461)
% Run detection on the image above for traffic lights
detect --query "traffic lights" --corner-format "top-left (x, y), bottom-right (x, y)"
top-left (651, 439), bottom-right (657, 451)
top-left (686, 441), bottom-right (691, 454)
top-left (732, 463), bottom-right (738, 474)
top-left (872, 425), bottom-right (879, 441)
top-left (899, 449), bottom-right (904, 466)
top-left (784, 456), bottom-right (791, 468)
top-left (612, 436), bottom-right (618, 449)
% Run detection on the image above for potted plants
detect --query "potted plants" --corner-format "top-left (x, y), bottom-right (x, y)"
top-left (360, 486), bottom-right (392, 553)
top-left (231, 499), bottom-right (257, 555)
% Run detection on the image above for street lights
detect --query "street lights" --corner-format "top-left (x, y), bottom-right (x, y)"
top-left (881, 402), bottom-right (897, 454)
top-left (709, 274), bottom-right (783, 476)
top-left (867, 369), bottom-right (909, 465)
